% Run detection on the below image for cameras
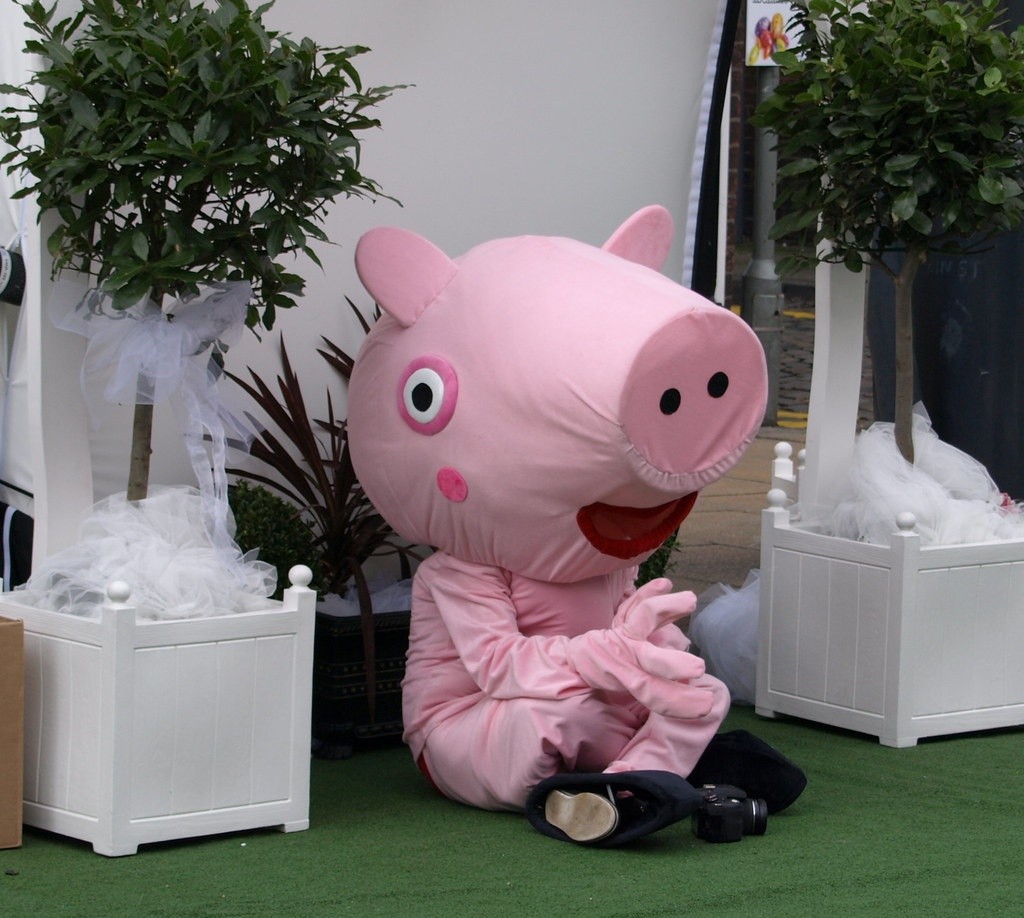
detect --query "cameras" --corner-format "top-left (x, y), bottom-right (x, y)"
top-left (692, 783), bottom-right (767, 843)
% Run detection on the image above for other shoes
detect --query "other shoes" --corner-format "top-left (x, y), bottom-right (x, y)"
top-left (546, 790), bottom-right (619, 843)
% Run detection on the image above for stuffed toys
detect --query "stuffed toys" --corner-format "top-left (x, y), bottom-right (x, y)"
top-left (348, 205), bottom-right (807, 842)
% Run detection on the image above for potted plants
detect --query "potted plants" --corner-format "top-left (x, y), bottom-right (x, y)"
top-left (754, 1), bottom-right (1024, 746)
top-left (1, 0), bottom-right (415, 854)
